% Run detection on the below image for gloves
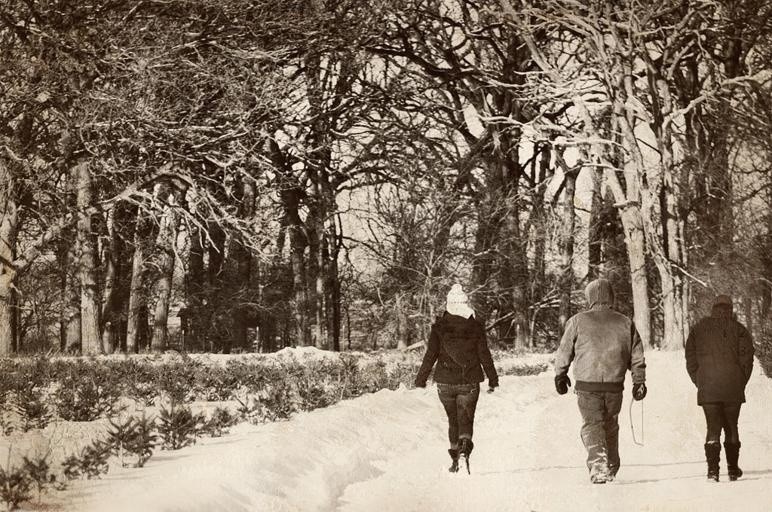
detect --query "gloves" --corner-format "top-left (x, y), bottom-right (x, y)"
top-left (632, 384), bottom-right (648, 401)
top-left (555, 373), bottom-right (571, 394)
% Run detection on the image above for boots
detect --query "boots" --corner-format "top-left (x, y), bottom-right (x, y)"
top-left (447, 437), bottom-right (474, 476)
top-left (705, 442), bottom-right (721, 482)
top-left (723, 441), bottom-right (742, 481)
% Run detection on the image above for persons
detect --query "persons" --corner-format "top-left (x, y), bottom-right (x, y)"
top-left (411, 282), bottom-right (498, 480)
top-left (684, 294), bottom-right (755, 482)
top-left (551, 277), bottom-right (648, 484)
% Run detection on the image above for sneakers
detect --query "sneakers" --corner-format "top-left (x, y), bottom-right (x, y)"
top-left (593, 468), bottom-right (618, 484)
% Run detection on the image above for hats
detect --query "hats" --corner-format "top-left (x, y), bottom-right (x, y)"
top-left (711, 295), bottom-right (733, 307)
top-left (446, 283), bottom-right (468, 306)
